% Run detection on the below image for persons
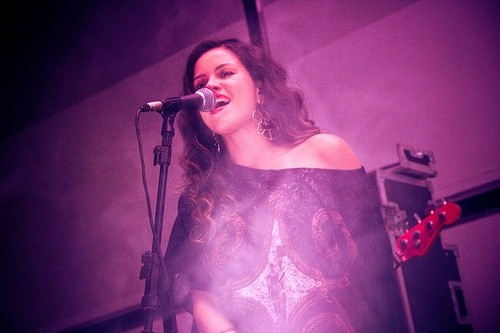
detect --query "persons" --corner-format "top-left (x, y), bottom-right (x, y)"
top-left (164, 37), bottom-right (394, 333)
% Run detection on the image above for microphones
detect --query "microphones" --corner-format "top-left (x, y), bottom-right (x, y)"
top-left (141, 88), bottom-right (216, 113)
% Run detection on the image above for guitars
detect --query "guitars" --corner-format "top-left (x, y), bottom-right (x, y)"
top-left (391, 201), bottom-right (462, 271)
